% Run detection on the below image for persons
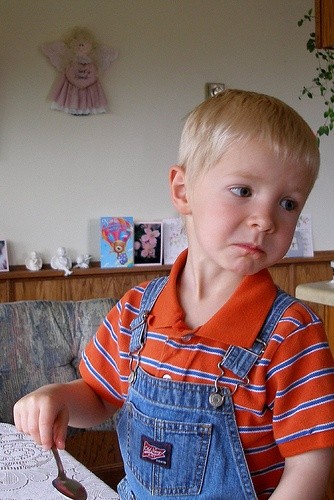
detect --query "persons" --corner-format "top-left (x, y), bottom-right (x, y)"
top-left (56, 246), bottom-right (73, 277)
top-left (14, 86), bottom-right (334, 500)
top-left (30, 250), bottom-right (40, 271)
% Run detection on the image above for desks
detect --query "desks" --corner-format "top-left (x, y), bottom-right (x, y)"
top-left (295, 280), bottom-right (334, 307)
top-left (0, 423), bottom-right (119, 500)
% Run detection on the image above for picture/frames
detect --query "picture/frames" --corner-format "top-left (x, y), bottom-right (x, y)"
top-left (133, 221), bottom-right (162, 266)
top-left (0, 239), bottom-right (9, 272)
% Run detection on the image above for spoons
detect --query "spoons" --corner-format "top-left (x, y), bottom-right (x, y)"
top-left (51, 438), bottom-right (87, 500)
